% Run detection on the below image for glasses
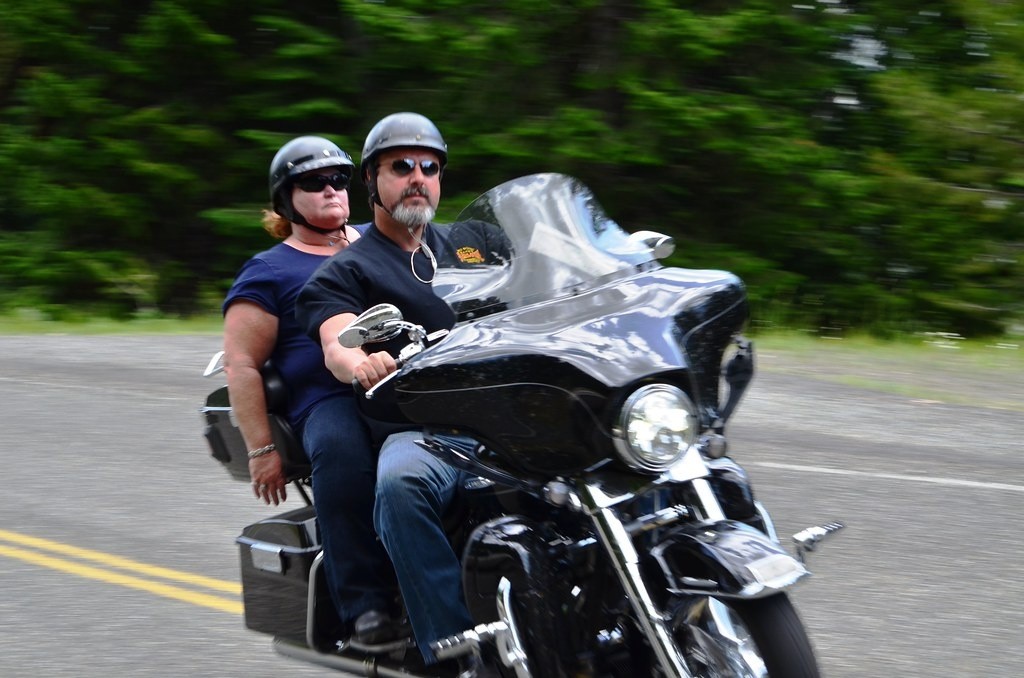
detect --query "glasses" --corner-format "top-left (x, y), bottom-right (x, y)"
top-left (380, 158), bottom-right (439, 176)
top-left (292, 173), bottom-right (352, 192)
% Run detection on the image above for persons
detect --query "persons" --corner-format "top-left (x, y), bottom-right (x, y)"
top-left (223, 112), bottom-right (515, 678)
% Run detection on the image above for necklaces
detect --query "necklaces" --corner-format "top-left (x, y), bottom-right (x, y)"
top-left (297, 237), bottom-right (341, 246)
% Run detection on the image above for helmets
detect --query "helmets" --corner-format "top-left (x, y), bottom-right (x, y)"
top-left (268, 134), bottom-right (355, 202)
top-left (358, 112), bottom-right (449, 168)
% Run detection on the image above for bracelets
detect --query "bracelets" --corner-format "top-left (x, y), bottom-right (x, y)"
top-left (248, 444), bottom-right (275, 459)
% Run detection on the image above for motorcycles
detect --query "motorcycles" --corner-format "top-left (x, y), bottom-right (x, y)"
top-left (194, 174), bottom-right (845, 675)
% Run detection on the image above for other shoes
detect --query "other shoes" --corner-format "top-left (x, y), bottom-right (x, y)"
top-left (346, 610), bottom-right (408, 651)
